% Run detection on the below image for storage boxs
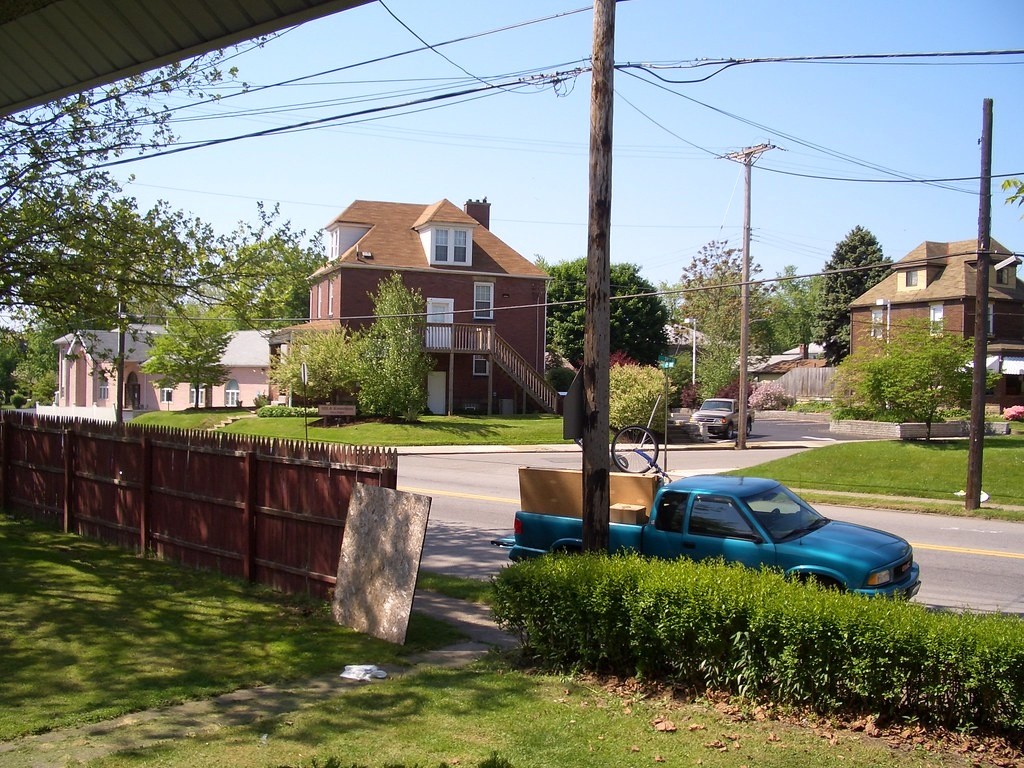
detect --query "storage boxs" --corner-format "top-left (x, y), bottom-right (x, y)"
top-left (610, 502), bottom-right (646, 525)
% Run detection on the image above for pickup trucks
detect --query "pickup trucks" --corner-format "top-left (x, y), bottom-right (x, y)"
top-left (489, 475), bottom-right (922, 605)
top-left (689, 398), bottom-right (756, 438)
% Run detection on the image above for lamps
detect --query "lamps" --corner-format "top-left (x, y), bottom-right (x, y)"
top-left (503, 294), bottom-right (509, 298)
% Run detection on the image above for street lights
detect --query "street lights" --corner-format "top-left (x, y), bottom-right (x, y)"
top-left (683, 318), bottom-right (697, 409)
top-left (876, 298), bottom-right (890, 408)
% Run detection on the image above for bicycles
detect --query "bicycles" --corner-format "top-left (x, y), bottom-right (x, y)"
top-left (610, 425), bottom-right (673, 485)
